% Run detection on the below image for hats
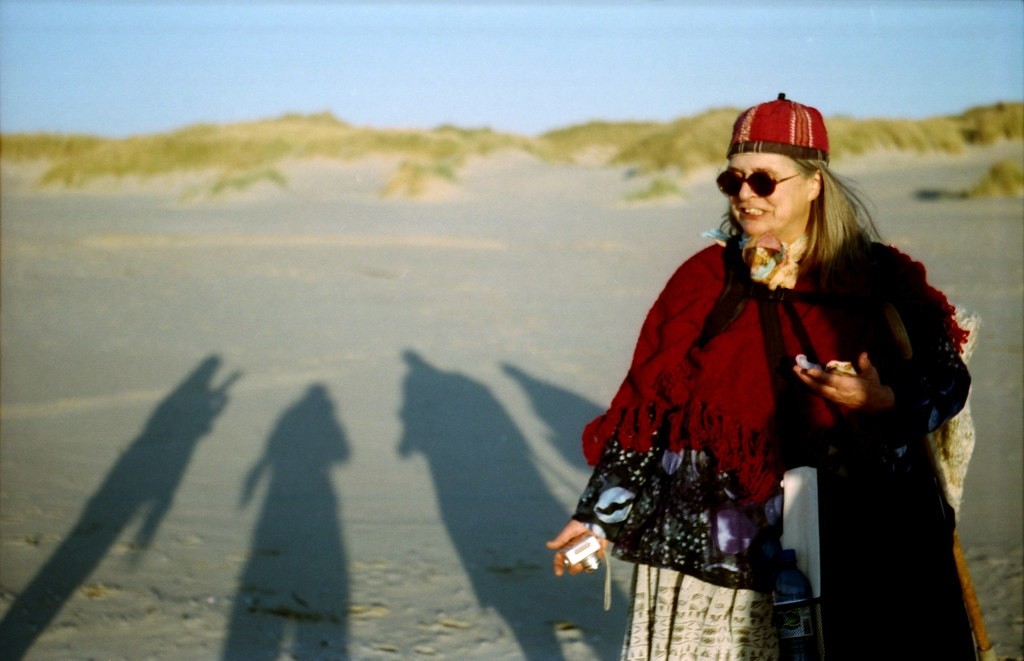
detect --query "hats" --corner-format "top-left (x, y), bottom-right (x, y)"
top-left (727, 92), bottom-right (829, 166)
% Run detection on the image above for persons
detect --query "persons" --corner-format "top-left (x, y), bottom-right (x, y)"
top-left (546, 93), bottom-right (995, 661)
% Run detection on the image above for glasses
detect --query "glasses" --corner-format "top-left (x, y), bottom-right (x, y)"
top-left (716, 169), bottom-right (810, 198)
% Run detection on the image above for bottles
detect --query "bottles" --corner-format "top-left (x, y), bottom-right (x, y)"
top-left (771, 549), bottom-right (821, 661)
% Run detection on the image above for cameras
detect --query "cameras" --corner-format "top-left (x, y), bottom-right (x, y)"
top-left (559, 534), bottom-right (602, 573)
